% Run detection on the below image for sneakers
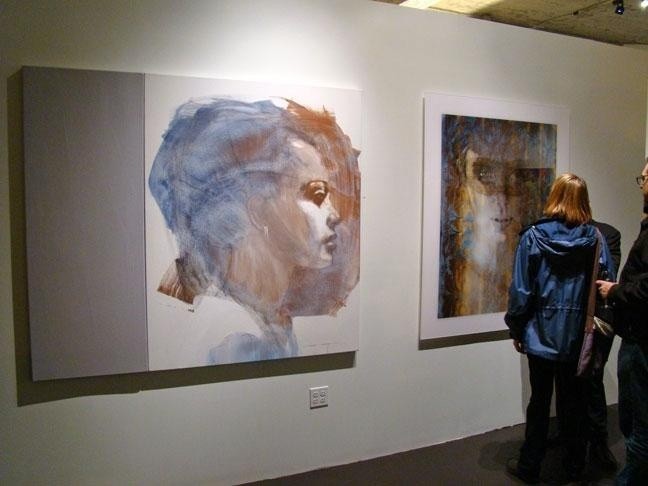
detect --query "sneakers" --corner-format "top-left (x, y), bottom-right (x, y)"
top-left (506, 456), bottom-right (539, 484)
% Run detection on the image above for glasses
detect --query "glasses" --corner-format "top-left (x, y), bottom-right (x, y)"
top-left (636, 175), bottom-right (648, 185)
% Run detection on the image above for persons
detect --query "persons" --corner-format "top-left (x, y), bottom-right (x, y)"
top-left (550, 211), bottom-right (623, 476)
top-left (150, 100), bottom-right (341, 312)
top-left (457, 120), bottom-right (536, 247)
top-left (500, 172), bottom-right (616, 486)
top-left (591, 157), bottom-right (648, 486)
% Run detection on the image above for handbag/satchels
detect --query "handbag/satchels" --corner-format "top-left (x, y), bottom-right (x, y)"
top-left (576, 315), bottom-right (614, 377)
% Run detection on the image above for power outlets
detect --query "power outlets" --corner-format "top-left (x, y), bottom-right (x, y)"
top-left (310, 386), bottom-right (329, 409)
